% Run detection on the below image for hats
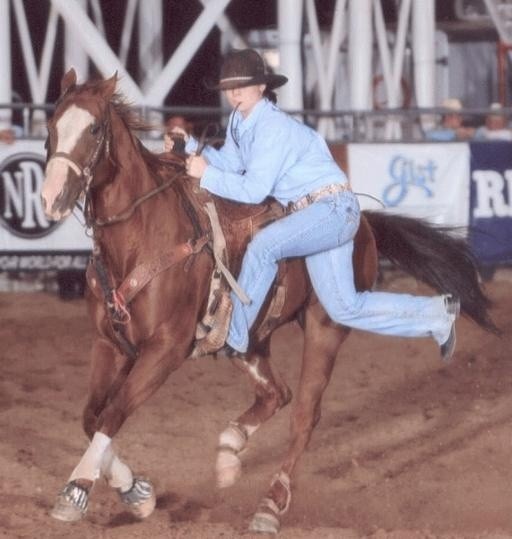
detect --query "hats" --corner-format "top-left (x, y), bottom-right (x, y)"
top-left (202, 48), bottom-right (287, 92)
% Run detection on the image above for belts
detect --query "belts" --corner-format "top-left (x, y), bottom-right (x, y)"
top-left (289, 182), bottom-right (351, 213)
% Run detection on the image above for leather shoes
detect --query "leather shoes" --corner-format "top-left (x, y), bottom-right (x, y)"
top-left (197, 343), bottom-right (249, 361)
top-left (440, 295), bottom-right (460, 359)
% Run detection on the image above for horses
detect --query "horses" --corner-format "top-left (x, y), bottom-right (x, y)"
top-left (38, 66), bottom-right (505, 538)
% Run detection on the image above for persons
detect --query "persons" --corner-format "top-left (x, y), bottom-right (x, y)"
top-left (163, 48), bottom-right (463, 363)
top-left (1, 124), bottom-right (23, 141)
top-left (472, 101), bottom-right (510, 143)
top-left (422, 99), bottom-right (476, 140)
